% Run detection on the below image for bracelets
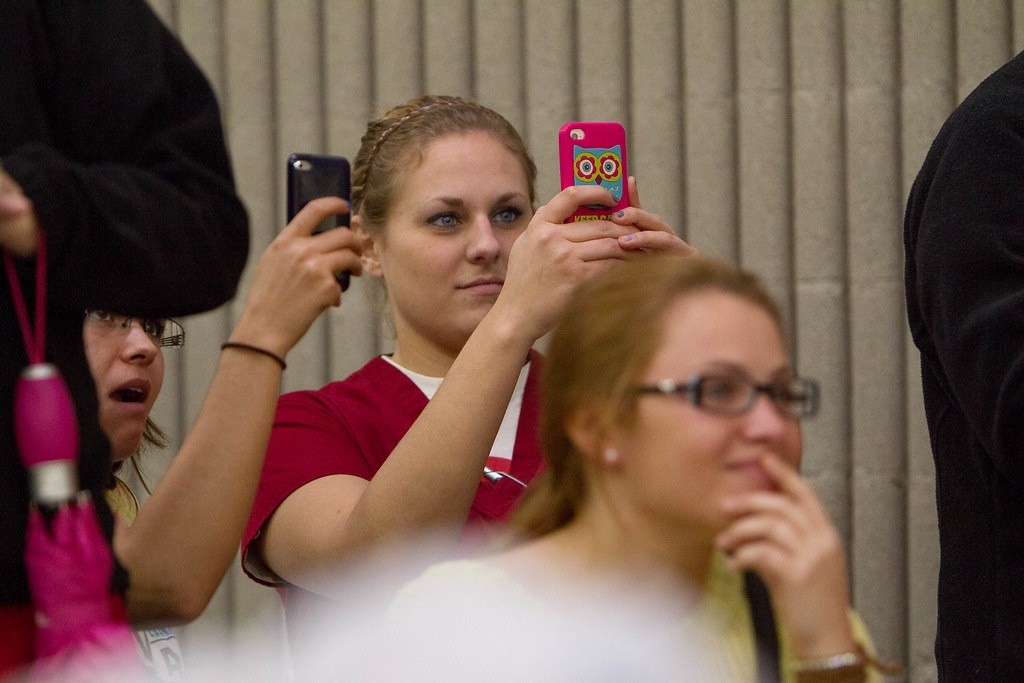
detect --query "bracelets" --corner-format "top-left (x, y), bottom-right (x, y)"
top-left (792, 654), bottom-right (866, 683)
top-left (218, 340), bottom-right (288, 370)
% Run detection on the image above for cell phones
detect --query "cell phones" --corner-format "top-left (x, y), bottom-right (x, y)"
top-left (284, 152), bottom-right (352, 292)
top-left (558, 122), bottom-right (630, 224)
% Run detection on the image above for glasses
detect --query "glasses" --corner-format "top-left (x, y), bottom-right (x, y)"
top-left (636, 373), bottom-right (819, 418)
top-left (84, 307), bottom-right (186, 353)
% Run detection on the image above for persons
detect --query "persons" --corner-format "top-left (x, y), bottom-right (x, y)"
top-left (1, 1), bottom-right (372, 682)
top-left (388, 253), bottom-right (886, 683)
top-left (240, 96), bottom-right (697, 682)
top-left (901, 48), bottom-right (1024, 683)
